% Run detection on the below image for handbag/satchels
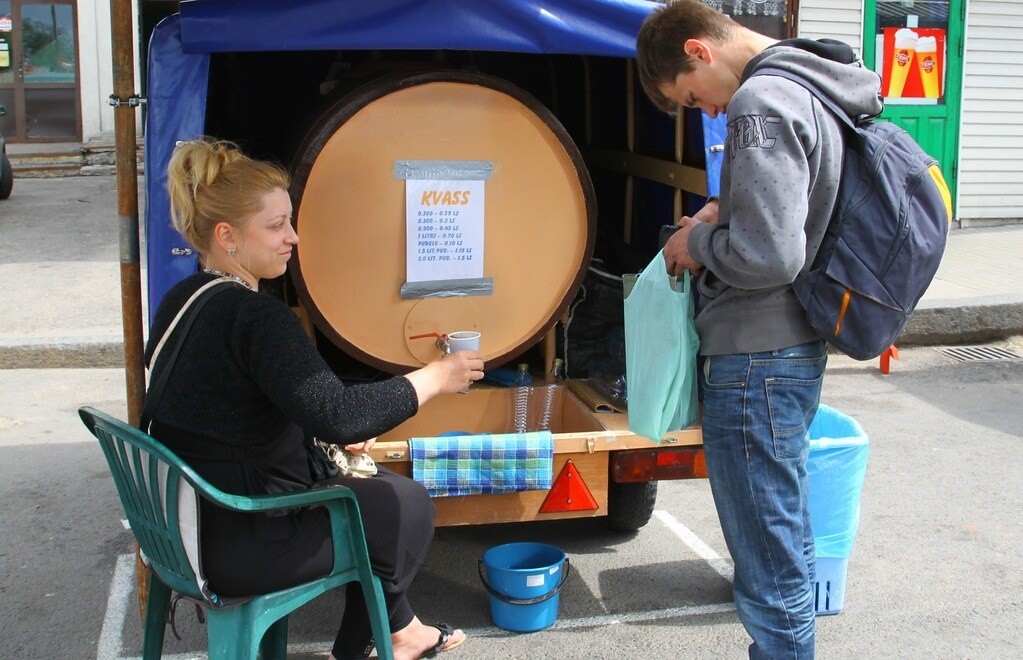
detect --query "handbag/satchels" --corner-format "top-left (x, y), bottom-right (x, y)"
top-left (316, 441), bottom-right (378, 479)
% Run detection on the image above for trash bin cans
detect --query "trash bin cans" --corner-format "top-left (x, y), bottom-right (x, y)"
top-left (804, 402), bottom-right (868, 617)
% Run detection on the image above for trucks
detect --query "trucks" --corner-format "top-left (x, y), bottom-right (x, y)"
top-left (141, 0), bottom-right (770, 534)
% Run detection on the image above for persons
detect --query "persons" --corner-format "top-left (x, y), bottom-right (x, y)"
top-left (139, 135), bottom-right (467, 660)
top-left (634, 0), bottom-right (884, 659)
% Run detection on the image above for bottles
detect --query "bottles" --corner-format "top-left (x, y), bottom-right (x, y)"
top-left (535, 359), bottom-right (565, 432)
top-left (507, 363), bottom-right (538, 434)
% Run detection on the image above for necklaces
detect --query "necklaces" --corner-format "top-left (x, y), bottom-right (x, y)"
top-left (202, 268), bottom-right (259, 293)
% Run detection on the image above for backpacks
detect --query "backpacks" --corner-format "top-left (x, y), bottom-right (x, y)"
top-left (750, 68), bottom-right (951, 360)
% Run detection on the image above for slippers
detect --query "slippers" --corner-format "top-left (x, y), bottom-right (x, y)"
top-left (414, 625), bottom-right (465, 660)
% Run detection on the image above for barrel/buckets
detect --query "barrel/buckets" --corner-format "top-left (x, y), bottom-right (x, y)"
top-left (478, 540), bottom-right (570, 635)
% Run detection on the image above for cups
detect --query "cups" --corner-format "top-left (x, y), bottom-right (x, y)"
top-left (447, 329), bottom-right (481, 357)
top-left (886, 35), bottom-right (917, 97)
top-left (914, 40), bottom-right (940, 98)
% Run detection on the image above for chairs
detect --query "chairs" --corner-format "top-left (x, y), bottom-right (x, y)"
top-left (80, 404), bottom-right (393, 660)
